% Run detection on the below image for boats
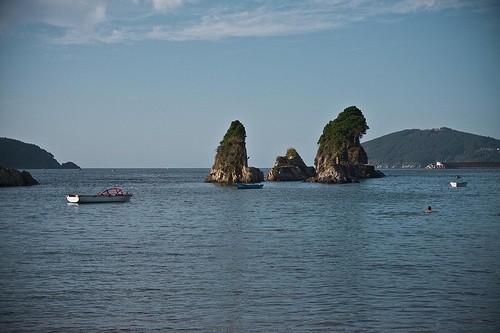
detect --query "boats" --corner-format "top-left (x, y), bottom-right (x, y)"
top-left (450, 175), bottom-right (467, 187)
top-left (63, 188), bottom-right (132, 203)
top-left (235, 182), bottom-right (264, 189)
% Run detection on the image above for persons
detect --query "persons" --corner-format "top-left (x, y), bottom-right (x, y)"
top-left (423, 205), bottom-right (433, 214)
